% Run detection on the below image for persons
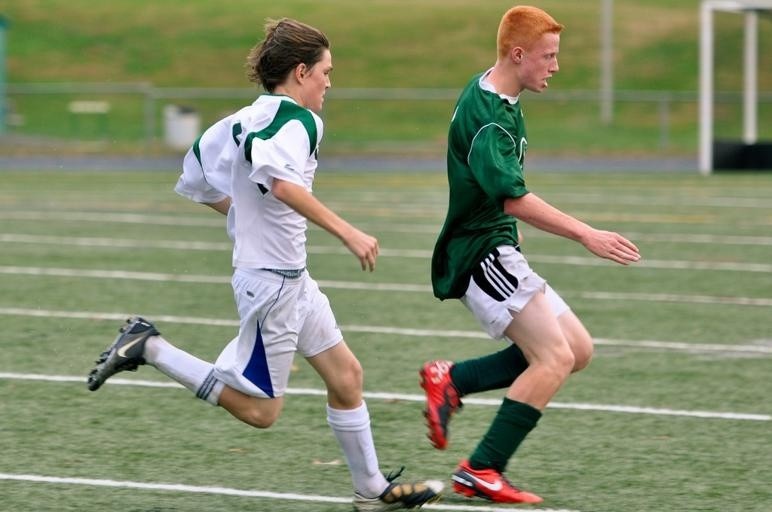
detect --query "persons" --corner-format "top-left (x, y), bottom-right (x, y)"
top-left (418, 4), bottom-right (642, 504)
top-left (88, 17), bottom-right (445, 512)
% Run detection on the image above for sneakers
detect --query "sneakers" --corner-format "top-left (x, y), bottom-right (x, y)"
top-left (351, 466), bottom-right (445, 512)
top-left (450, 459), bottom-right (543, 504)
top-left (418, 360), bottom-right (463, 451)
top-left (87, 317), bottom-right (160, 391)
